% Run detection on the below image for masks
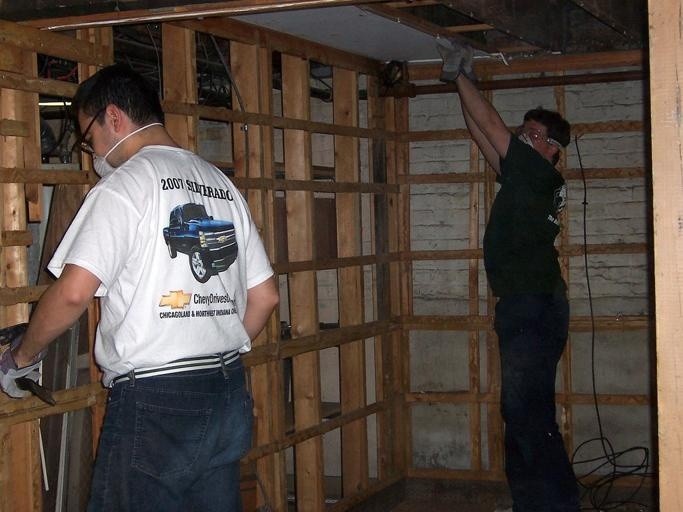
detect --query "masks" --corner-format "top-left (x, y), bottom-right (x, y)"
top-left (90, 152), bottom-right (117, 179)
top-left (517, 131), bottom-right (533, 151)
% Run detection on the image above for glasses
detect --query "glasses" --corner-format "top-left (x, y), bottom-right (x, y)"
top-left (517, 123), bottom-right (565, 152)
top-left (77, 106), bottom-right (106, 155)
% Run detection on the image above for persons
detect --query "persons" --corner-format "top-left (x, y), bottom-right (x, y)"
top-left (0, 62), bottom-right (284, 511)
top-left (431, 32), bottom-right (587, 510)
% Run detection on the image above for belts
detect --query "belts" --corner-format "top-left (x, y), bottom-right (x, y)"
top-left (109, 347), bottom-right (241, 385)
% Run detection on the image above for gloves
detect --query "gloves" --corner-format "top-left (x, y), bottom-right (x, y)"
top-left (435, 39), bottom-right (477, 86)
top-left (0, 332), bottom-right (49, 400)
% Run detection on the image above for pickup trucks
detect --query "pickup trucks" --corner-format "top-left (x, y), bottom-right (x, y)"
top-left (161, 201), bottom-right (238, 283)
